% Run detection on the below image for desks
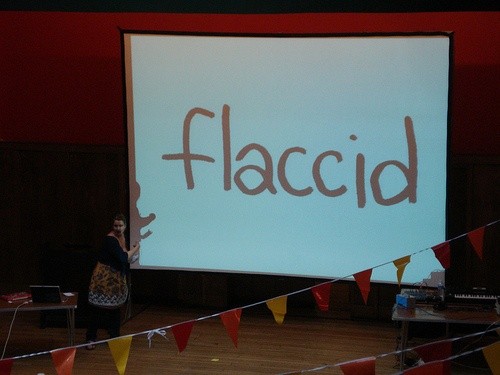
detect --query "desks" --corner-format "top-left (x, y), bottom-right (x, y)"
top-left (392, 303), bottom-right (500, 371)
top-left (0, 293), bottom-right (78, 349)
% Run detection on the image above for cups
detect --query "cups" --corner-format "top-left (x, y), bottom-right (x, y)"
top-left (432, 296), bottom-right (442, 311)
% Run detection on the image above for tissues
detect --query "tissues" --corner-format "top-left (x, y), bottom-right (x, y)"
top-left (396, 294), bottom-right (416, 308)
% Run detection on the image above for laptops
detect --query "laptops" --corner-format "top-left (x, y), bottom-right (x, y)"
top-left (28, 285), bottom-right (68, 304)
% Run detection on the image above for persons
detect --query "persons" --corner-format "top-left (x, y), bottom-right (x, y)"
top-left (85, 215), bottom-right (139, 350)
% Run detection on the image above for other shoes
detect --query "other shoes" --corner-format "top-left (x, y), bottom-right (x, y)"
top-left (87, 340), bottom-right (97, 349)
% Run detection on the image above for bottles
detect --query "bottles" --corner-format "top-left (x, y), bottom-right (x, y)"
top-left (437, 279), bottom-right (445, 300)
